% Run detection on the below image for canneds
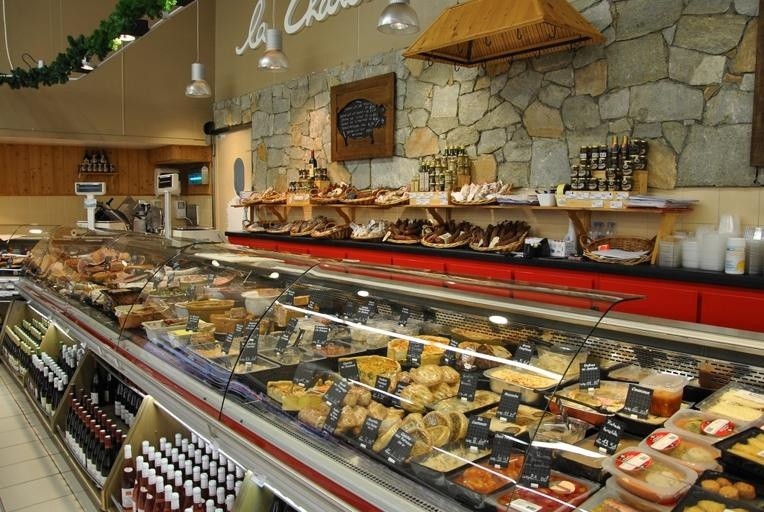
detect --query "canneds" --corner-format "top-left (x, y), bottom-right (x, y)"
top-left (81, 163), bottom-right (115, 172)
top-left (435, 157), bottom-right (471, 192)
top-left (571, 140), bottom-right (648, 192)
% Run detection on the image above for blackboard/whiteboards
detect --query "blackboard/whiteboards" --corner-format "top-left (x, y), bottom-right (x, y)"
top-left (330, 71), bottom-right (396, 162)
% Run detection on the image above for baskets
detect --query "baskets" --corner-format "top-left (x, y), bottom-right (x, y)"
top-left (580, 237), bottom-right (655, 265)
top-left (66, 251), bottom-right (309, 335)
top-left (242, 219), bottom-right (530, 254)
top-left (239, 181), bottom-right (512, 205)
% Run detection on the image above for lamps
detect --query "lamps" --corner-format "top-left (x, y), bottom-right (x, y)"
top-left (186, 0), bottom-right (290, 100)
top-left (376, 0), bottom-right (420, 36)
top-left (119, 17), bottom-right (150, 42)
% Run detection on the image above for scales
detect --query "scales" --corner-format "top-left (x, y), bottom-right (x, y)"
top-left (157, 174), bottom-right (228, 243)
top-left (74, 181), bottom-right (126, 231)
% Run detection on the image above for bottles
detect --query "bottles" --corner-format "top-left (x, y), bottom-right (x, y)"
top-left (1, 314), bottom-right (247, 512)
top-left (201, 165), bottom-right (209, 185)
top-left (571, 135), bottom-right (648, 190)
top-left (724, 238), bottom-right (745, 275)
top-left (589, 220), bottom-right (616, 241)
top-left (288, 149), bottom-right (329, 194)
top-left (79, 151), bottom-right (114, 173)
top-left (410, 146), bottom-right (470, 192)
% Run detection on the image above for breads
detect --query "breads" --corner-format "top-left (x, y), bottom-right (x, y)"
top-left (267, 335), bottom-right (513, 462)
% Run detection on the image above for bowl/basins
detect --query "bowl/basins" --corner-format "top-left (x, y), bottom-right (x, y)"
top-left (537, 193), bottom-right (555, 208)
top-left (243, 287), bottom-right (285, 315)
top-left (188, 299), bottom-right (254, 330)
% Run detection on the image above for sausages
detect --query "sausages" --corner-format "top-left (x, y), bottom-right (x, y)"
top-left (388, 218), bottom-right (530, 246)
